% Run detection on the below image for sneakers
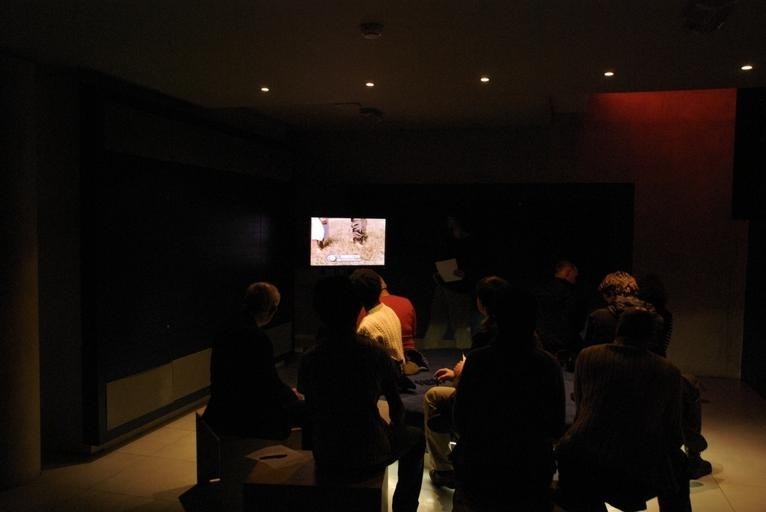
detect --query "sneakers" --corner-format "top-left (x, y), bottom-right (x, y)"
top-left (430, 469), bottom-right (456, 489)
top-left (688, 460), bottom-right (711, 480)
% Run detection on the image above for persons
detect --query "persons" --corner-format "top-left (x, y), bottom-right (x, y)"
top-left (427, 285), bottom-right (567, 509)
top-left (298, 269), bottom-right (426, 510)
top-left (574, 307), bottom-right (702, 510)
top-left (314, 217), bottom-right (369, 245)
top-left (426, 211), bottom-right (492, 343)
top-left (204, 279), bottom-right (305, 437)
top-left (353, 263), bottom-right (656, 479)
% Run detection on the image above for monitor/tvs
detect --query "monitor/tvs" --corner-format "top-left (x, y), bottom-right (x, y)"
top-left (308, 214), bottom-right (388, 270)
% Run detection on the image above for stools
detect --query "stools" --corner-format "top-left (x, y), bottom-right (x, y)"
top-left (399, 347), bottom-right (575, 466)
top-left (195, 399), bottom-right (391, 511)
